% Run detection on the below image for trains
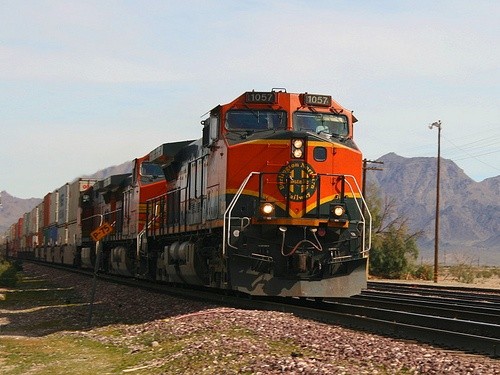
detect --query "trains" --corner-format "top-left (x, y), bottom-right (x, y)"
top-left (7, 87), bottom-right (372, 298)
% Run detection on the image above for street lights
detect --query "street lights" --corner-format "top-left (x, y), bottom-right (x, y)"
top-left (430, 119), bottom-right (442, 281)
